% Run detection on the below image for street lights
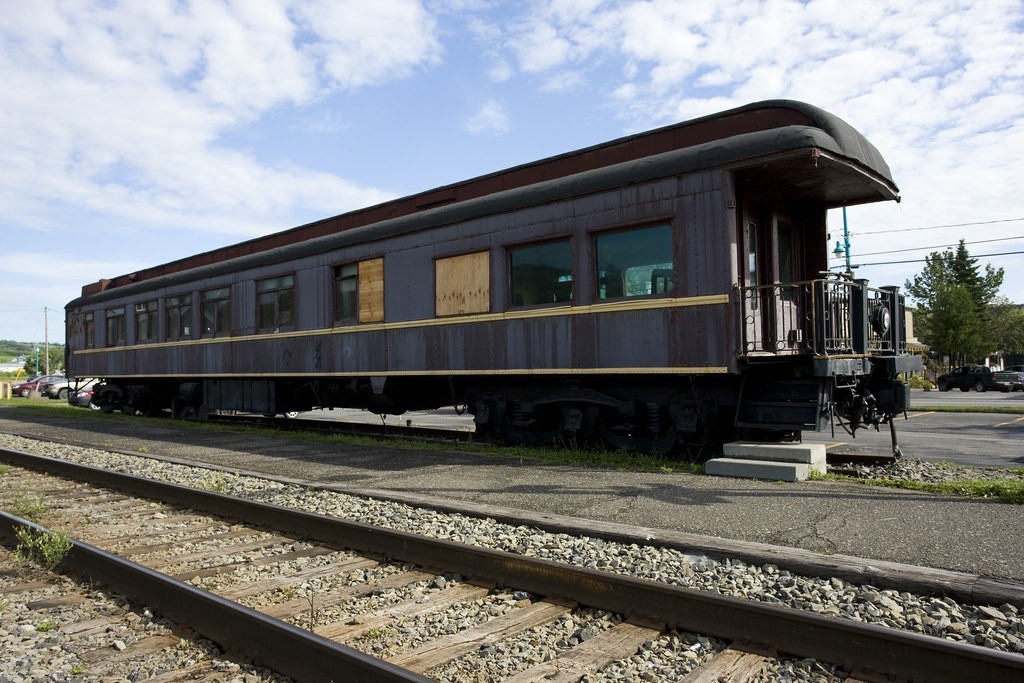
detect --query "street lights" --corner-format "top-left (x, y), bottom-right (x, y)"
top-left (34, 346), bottom-right (39, 375)
top-left (832, 207), bottom-right (852, 271)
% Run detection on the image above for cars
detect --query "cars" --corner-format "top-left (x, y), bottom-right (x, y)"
top-left (44, 377), bottom-right (107, 401)
top-left (69, 385), bottom-right (101, 410)
top-left (12, 374), bottom-right (65, 396)
top-left (1003, 364), bottom-right (1024, 392)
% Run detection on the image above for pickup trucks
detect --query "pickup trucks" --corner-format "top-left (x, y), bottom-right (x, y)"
top-left (936, 362), bottom-right (1019, 394)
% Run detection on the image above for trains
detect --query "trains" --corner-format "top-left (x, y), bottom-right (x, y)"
top-left (63, 99), bottom-right (926, 467)
